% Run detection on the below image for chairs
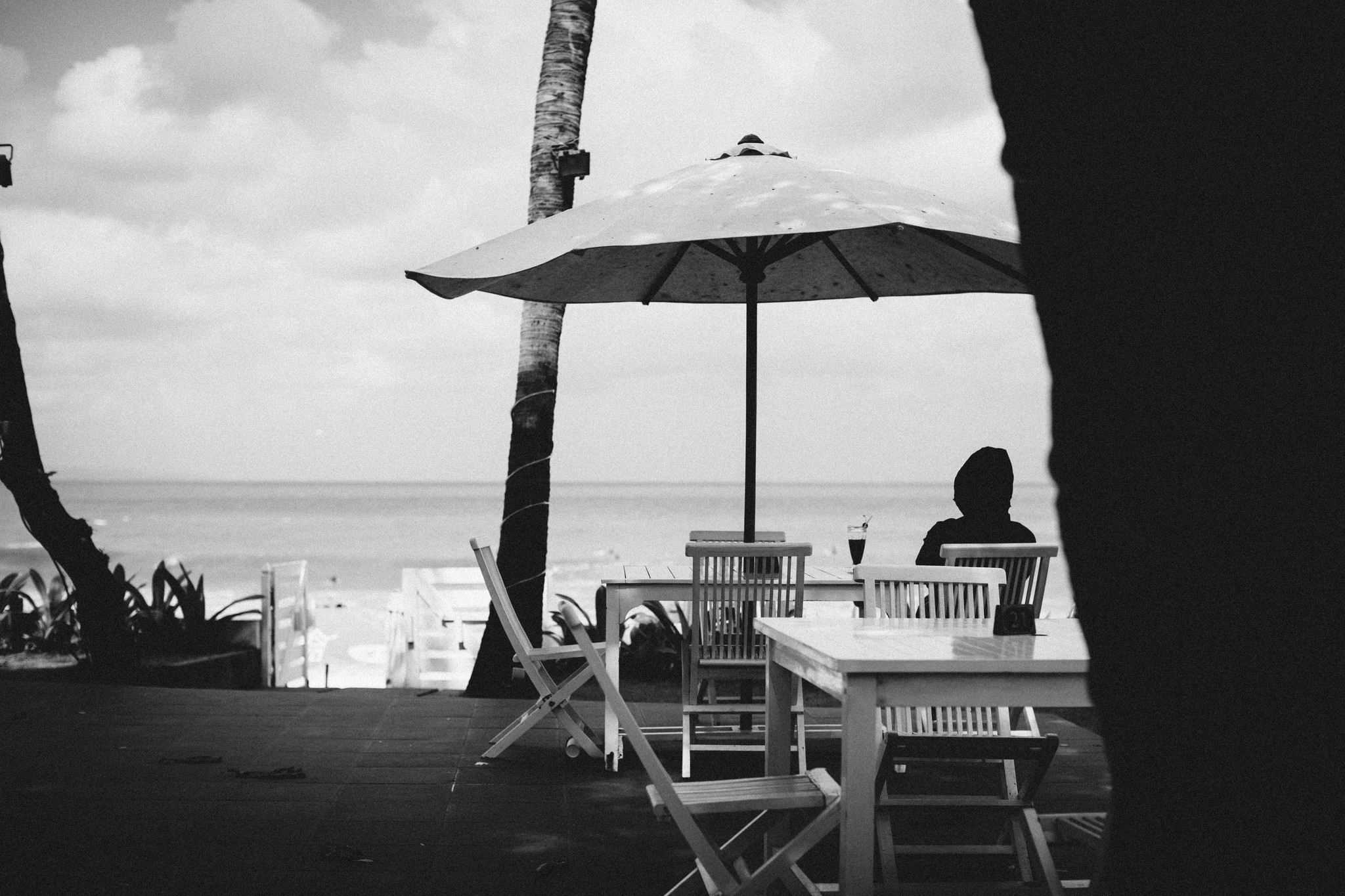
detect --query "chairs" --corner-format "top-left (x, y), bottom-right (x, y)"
top-left (465, 524), bottom-right (1095, 896)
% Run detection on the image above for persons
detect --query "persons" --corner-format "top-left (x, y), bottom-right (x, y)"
top-left (917, 448), bottom-right (1038, 723)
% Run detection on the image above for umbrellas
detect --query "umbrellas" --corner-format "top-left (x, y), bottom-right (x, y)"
top-left (404, 137), bottom-right (1035, 730)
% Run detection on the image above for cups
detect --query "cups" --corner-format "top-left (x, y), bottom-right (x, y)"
top-left (847, 525), bottom-right (866, 573)
